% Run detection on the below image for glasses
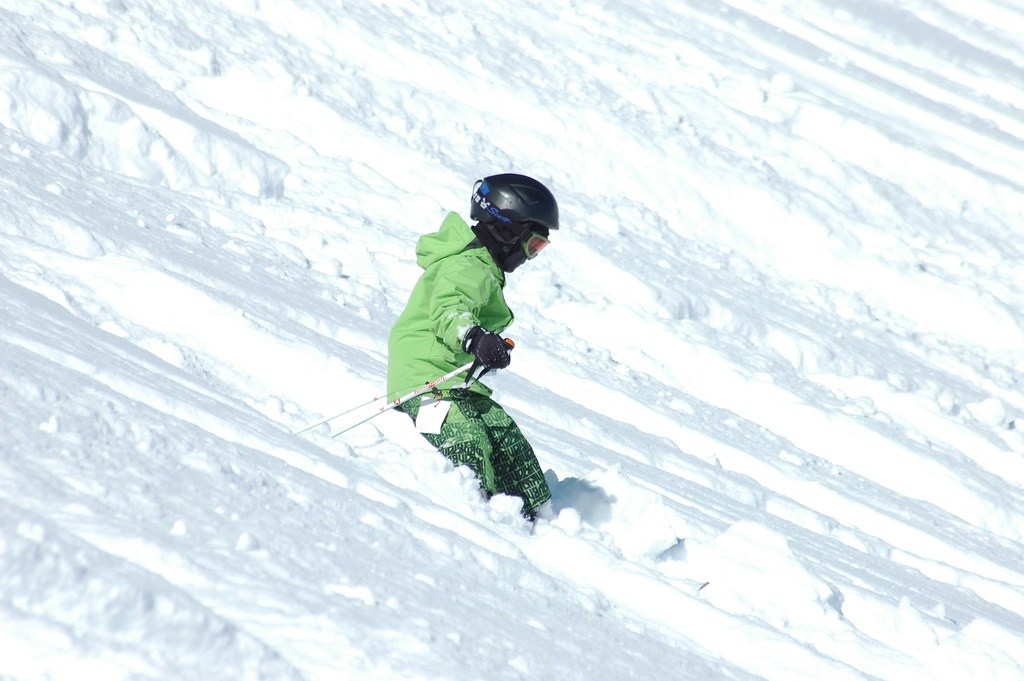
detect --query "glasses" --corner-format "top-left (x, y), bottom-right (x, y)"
top-left (521, 230), bottom-right (550, 260)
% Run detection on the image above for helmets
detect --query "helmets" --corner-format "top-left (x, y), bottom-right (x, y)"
top-left (470, 173), bottom-right (560, 245)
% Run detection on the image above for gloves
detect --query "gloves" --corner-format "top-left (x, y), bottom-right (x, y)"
top-left (462, 325), bottom-right (511, 371)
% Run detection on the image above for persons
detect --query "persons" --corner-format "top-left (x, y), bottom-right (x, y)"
top-left (386, 173), bottom-right (559, 522)
